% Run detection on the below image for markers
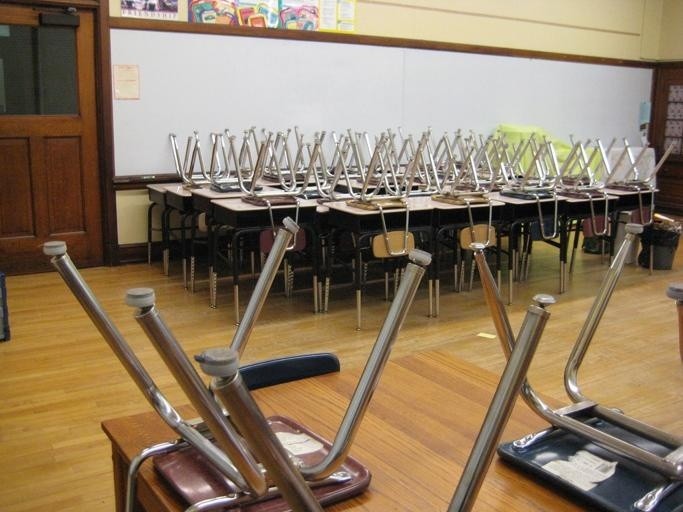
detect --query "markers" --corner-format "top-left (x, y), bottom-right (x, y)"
top-left (142, 176), bottom-right (155, 180)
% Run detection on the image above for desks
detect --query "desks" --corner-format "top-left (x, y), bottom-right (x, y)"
top-left (143, 175), bottom-right (661, 332)
top-left (97, 347), bottom-right (682, 511)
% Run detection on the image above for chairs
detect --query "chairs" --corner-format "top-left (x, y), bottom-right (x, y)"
top-left (168, 124), bottom-right (658, 260)
top-left (40, 220), bottom-right (683, 510)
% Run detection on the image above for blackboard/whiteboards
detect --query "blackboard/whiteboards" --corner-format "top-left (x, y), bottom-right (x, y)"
top-left (101, 17), bottom-right (657, 190)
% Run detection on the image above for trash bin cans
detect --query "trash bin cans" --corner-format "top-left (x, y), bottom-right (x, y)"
top-left (640, 220), bottom-right (680, 270)
